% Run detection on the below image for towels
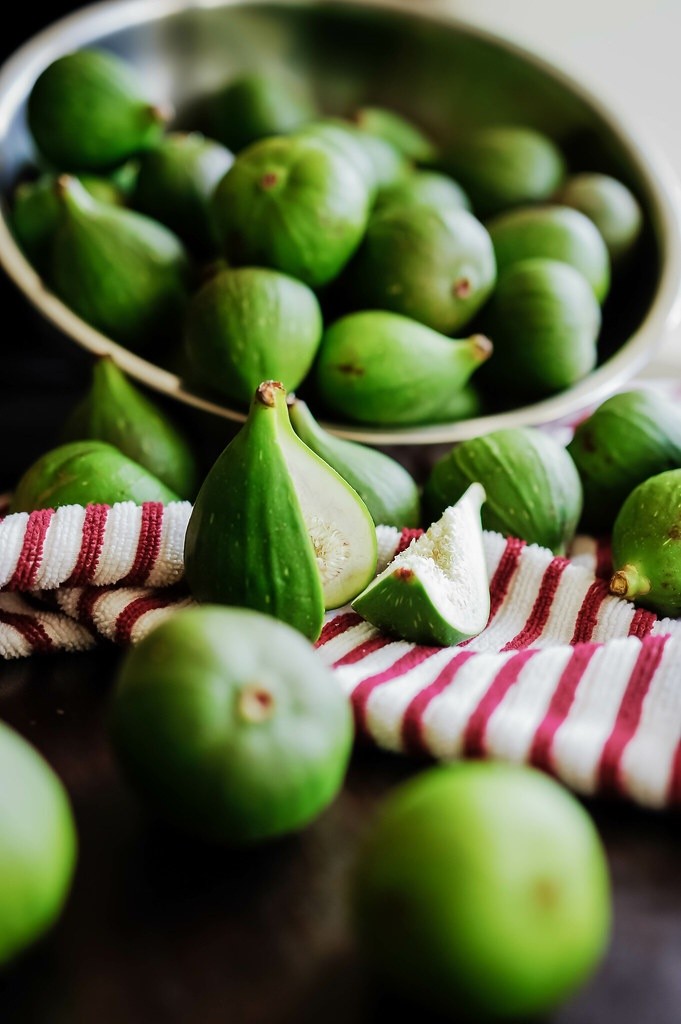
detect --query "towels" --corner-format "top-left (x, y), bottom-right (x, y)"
top-left (1, 499), bottom-right (680, 824)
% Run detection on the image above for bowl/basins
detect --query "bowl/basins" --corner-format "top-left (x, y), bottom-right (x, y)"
top-left (0, 0), bottom-right (679, 444)
